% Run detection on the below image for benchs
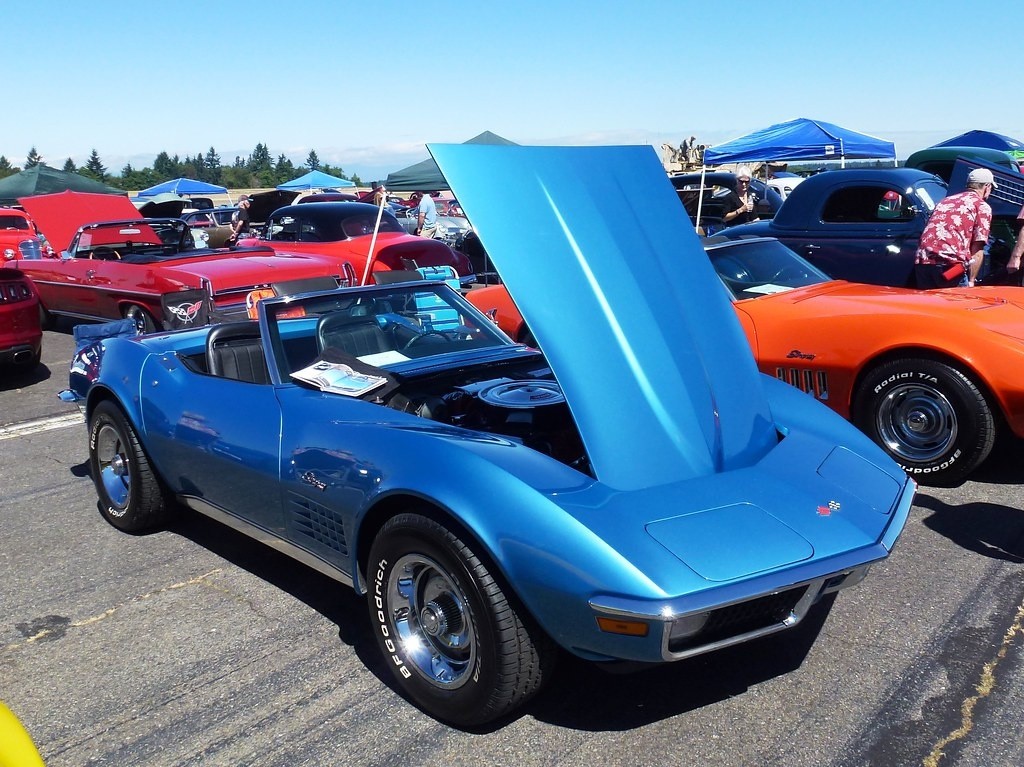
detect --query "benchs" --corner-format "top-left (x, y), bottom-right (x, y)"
top-left (122, 245), bottom-right (274, 267)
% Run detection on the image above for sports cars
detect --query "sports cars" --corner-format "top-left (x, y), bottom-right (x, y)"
top-left (0, 142), bottom-right (1024, 490)
top-left (58, 138), bottom-right (920, 728)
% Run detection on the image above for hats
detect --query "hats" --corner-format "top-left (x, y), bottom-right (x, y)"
top-left (967, 168), bottom-right (998, 188)
top-left (238, 195), bottom-right (254, 203)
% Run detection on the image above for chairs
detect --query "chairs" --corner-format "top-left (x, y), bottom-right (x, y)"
top-left (270, 276), bottom-right (339, 311)
top-left (315, 310), bottom-right (390, 370)
top-left (160, 287), bottom-right (222, 331)
top-left (373, 269), bottom-right (423, 285)
top-left (204, 322), bottom-right (272, 385)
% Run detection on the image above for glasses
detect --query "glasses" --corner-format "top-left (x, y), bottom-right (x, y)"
top-left (738, 179), bottom-right (750, 184)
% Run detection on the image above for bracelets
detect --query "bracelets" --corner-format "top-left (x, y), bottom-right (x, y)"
top-left (736, 209), bottom-right (739, 216)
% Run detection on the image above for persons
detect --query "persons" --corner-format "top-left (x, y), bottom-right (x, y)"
top-left (415, 190), bottom-right (437, 240)
top-left (680, 135), bottom-right (696, 161)
top-left (721, 165), bottom-right (760, 230)
top-left (1006, 204), bottom-right (1024, 274)
top-left (227, 195), bottom-right (253, 245)
top-left (373, 185), bottom-right (389, 210)
top-left (914, 167), bottom-right (999, 291)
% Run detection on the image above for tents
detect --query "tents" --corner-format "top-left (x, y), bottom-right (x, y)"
top-left (277, 169), bottom-right (361, 198)
top-left (137, 177), bottom-right (234, 208)
top-left (694, 116), bottom-right (899, 237)
top-left (0, 161), bottom-right (129, 208)
top-left (360, 130), bottom-right (524, 287)
top-left (924, 130), bottom-right (1024, 153)
